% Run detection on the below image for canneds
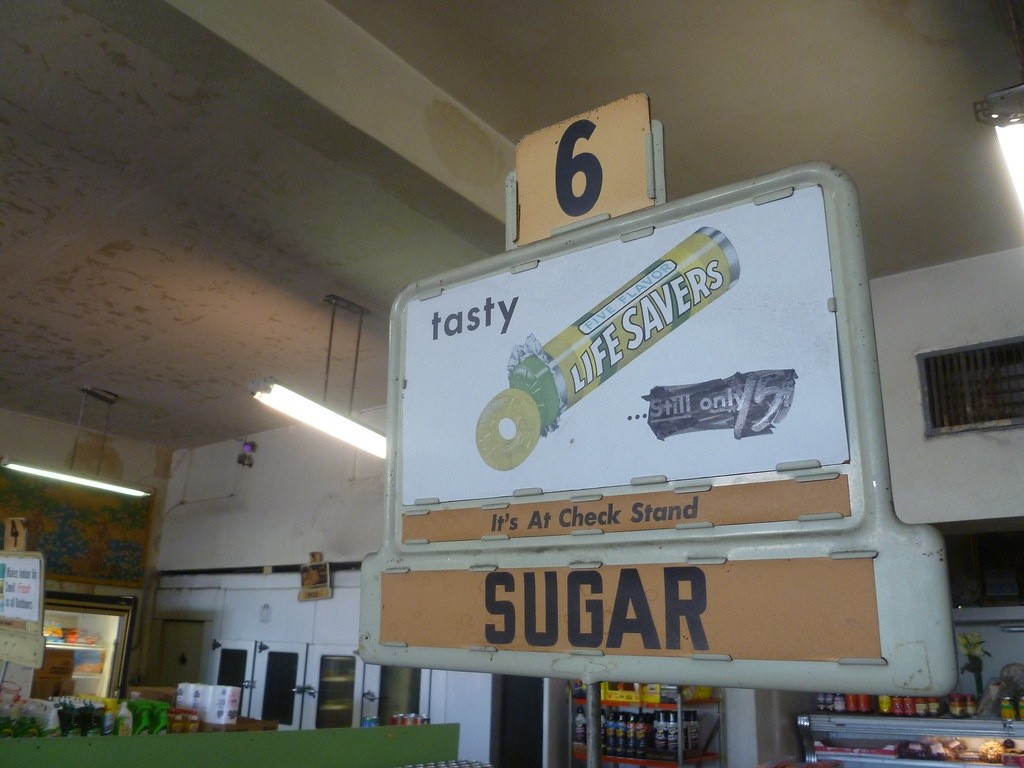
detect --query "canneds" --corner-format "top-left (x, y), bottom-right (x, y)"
top-left (403, 760), bottom-right (494, 768)
top-left (390, 713), bottom-right (431, 725)
top-left (878, 694), bottom-right (941, 717)
top-left (947, 693), bottom-right (977, 718)
top-left (362, 716), bottom-right (380, 727)
top-left (999, 695), bottom-right (1024, 721)
top-left (815, 692), bottom-right (874, 714)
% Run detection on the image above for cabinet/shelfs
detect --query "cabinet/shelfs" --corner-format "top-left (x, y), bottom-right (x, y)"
top-left (568, 687), bottom-right (726, 768)
top-left (795, 712), bottom-right (1024, 768)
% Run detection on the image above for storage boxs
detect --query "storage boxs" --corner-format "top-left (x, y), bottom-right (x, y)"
top-left (814, 741), bottom-right (900, 760)
top-left (176, 682), bottom-right (242, 724)
top-left (582, 681), bottom-right (713, 703)
top-left (32, 648), bottom-right (75, 700)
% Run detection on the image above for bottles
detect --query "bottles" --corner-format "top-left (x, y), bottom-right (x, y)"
top-left (48, 694), bottom-right (201, 738)
top-left (565, 679), bottom-right (700, 759)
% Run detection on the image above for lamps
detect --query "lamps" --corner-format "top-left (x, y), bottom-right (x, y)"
top-left (250, 293), bottom-right (389, 463)
top-left (0, 385), bottom-right (159, 501)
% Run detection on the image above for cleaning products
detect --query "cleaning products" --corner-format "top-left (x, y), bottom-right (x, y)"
top-left (0, 692), bottom-right (201, 739)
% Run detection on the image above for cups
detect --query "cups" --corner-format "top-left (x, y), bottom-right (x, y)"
top-left (816, 692), bottom-right (977, 719)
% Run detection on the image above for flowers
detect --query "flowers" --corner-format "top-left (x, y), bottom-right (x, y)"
top-left (957, 631), bottom-right (992, 697)
